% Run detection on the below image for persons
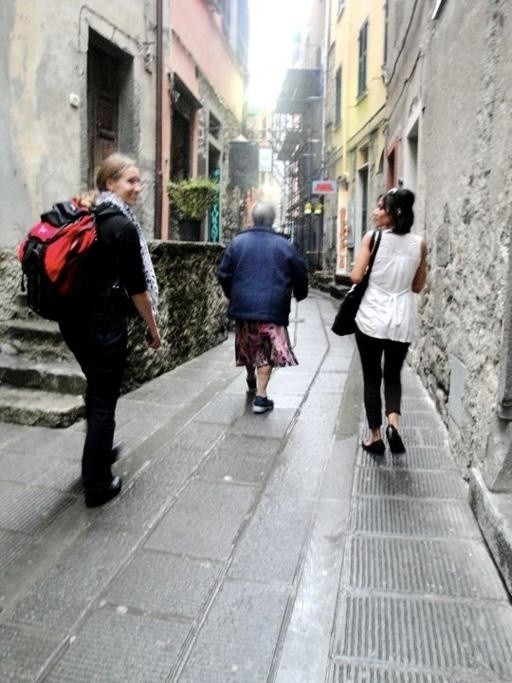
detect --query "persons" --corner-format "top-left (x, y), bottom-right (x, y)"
top-left (349, 187), bottom-right (429, 456)
top-left (57, 152), bottom-right (162, 508)
top-left (218, 202), bottom-right (309, 415)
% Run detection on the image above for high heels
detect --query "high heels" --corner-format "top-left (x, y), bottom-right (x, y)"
top-left (362, 439), bottom-right (386, 457)
top-left (386, 424), bottom-right (406, 455)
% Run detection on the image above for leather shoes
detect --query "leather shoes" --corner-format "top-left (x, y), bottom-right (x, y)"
top-left (85, 445), bottom-right (123, 508)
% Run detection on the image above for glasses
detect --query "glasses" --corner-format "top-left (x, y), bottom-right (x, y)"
top-left (388, 187), bottom-right (398, 195)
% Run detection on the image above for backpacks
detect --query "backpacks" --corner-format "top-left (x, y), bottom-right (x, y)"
top-left (17, 196), bottom-right (124, 324)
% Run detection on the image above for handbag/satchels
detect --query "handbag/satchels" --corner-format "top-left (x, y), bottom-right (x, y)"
top-left (331, 273), bottom-right (369, 337)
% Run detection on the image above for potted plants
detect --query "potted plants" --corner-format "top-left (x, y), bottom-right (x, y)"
top-left (166, 177), bottom-right (222, 240)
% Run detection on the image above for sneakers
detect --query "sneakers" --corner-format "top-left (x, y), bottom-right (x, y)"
top-left (245, 375), bottom-right (274, 414)
